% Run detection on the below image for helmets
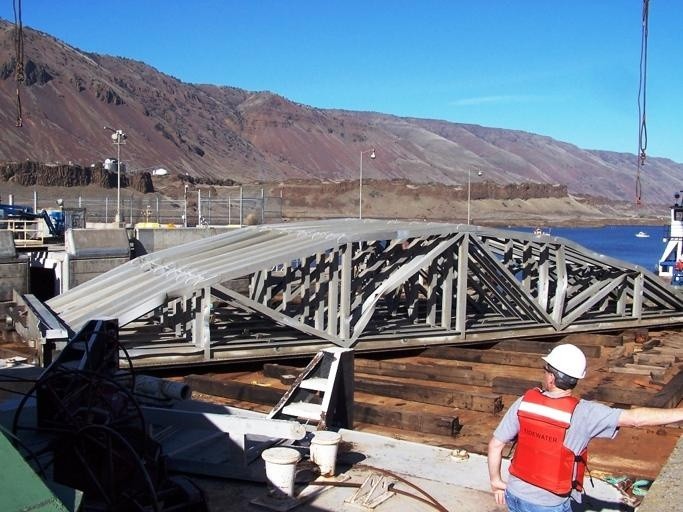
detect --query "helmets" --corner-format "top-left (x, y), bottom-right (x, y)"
top-left (541, 344), bottom-right (587, 380)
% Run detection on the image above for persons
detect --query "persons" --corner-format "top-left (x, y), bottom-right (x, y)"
top-left (488, 344), bottom-right (683, 511)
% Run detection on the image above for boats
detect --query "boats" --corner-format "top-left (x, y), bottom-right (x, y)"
top-left (636, 231), bottom-right (650, 238)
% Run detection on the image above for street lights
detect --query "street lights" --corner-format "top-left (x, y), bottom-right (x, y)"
top-left (467, 167), bottom-right (485, 228)
top-left (110, 129), bottom-right (127, 224)
top-left (359, 149), bottom-right (375, 218)
